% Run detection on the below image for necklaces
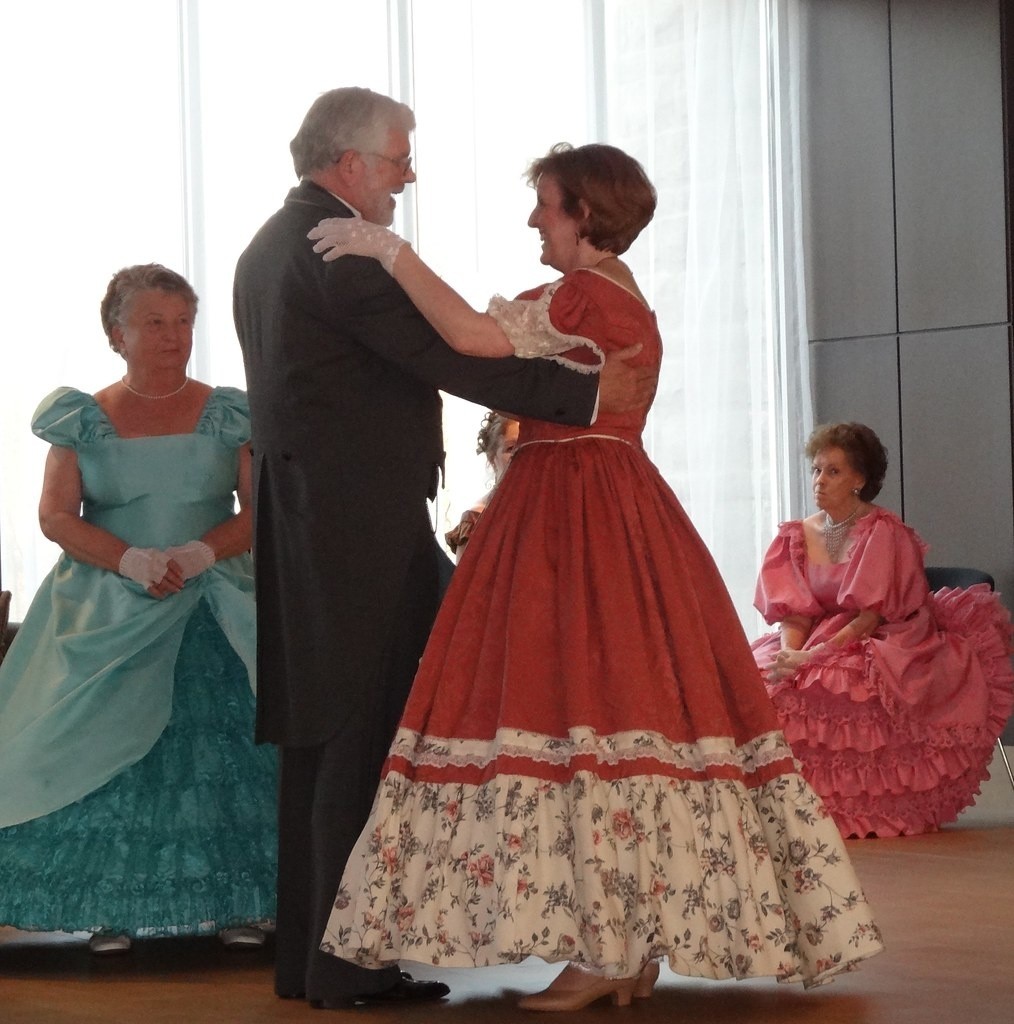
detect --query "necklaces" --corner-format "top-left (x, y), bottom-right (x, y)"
top-left (121, 375), bottom-right (189, 399)
top-left (823, 502), bottom-right (864, 564)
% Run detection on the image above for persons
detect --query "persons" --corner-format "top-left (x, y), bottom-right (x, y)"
top-left (751, 420), bottom-right (1014, 841)
top-left (445, 409), bottom-right (519, 568)
top-left (235, 86), bottom-right (660, 1008)
top-left (309, 143), bottom-right (883, 1012)
top-left (0, 265), bottom-right (280, 955)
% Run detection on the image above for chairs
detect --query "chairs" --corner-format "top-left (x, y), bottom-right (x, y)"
top-left (924, 566), bottom-right (1014, 792)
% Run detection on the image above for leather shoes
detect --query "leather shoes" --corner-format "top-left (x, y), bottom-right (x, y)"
top-left (217, 924), bottom-right (265, 948)
top-left (310, 976), bottom-right (450, 1009)
top-left (279, 971), bottom-right (414, 999)
top-left (88, 927), bottom-right (131, 956)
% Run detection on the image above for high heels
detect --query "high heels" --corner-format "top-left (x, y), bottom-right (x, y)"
top-left (632, 963), bottom-right (660, 998)
top-left (518, 975), bottom-right (638, 1011)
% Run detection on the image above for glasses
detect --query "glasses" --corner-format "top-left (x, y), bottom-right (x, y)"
top-left (337, 149), bottom-right (412, 176)
